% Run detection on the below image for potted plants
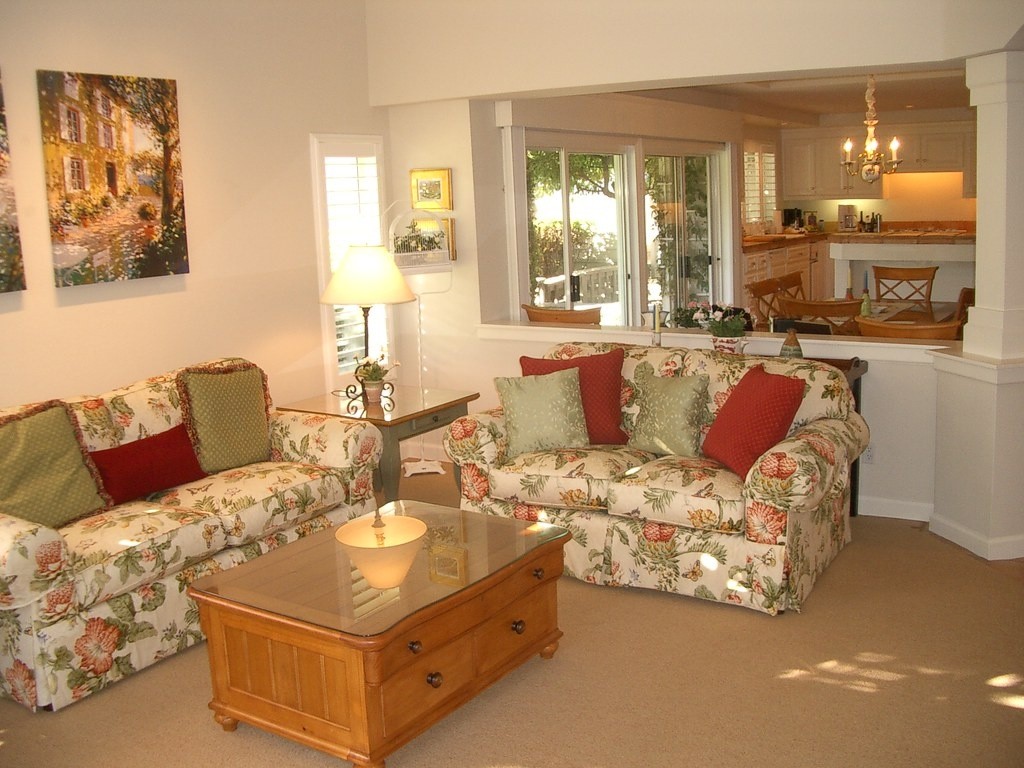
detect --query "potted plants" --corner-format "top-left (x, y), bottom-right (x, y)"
top-left (706, 312), bottom-right (747, 355)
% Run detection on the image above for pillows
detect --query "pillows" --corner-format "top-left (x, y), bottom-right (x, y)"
top-left (492, 348), bottom-right (805, 483)
top-left (0, 363), bottom-right (274, 526)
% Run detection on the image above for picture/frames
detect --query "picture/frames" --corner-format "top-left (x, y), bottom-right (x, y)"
top-left (412, 169), bottom-right (457, 260)
top-left (430, 546), bottom-right (467, 588)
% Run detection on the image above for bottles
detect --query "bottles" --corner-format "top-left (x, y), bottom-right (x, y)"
top-left (860, 288), bottom-right (871, 317)
top-left (794, 215), bottom-right (799, 230)
top-left (840, 221), bottom-right (845, 229)
top-left (846, 288), bottom-right (854, 301)
top-left (808, 212), bottom-right (816, 225)
top-left (859, 212), bottom-right (877, 232)
top-left (571, 270), bottom-right (581, 302)
top-left (819, 219), bottom-right (824, 231)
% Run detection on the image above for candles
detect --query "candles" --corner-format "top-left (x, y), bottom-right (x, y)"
top-left (864, 269), bottom-right (868, 290)
top-left (847, 266), bottom-right (854, 289)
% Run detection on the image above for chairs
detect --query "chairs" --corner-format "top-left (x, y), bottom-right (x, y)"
top-left (745, 266), bottom-right (975, 340)
top-left (522, 304), bottom-right (600, 326)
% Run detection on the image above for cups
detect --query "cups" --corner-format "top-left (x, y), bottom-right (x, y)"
top-left (845, 216), bottom-right (857, 228)
top-left (770, 226), bottom-right (784, 233)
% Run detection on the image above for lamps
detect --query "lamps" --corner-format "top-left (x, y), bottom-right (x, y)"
top-left (334, 506), bottom-right (427, 593)
top-left (319, 245), bottom-right (417, 400)
top-left (841, 74), bottom-right (903, 185)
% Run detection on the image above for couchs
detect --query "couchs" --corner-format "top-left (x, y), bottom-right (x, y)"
top-left (442, 342), bottom-right (871, 614)
top-left (0, 357), bottom-right (383, 712)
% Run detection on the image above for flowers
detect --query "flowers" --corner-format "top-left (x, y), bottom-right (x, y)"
top-left (354, 354), bottom-right (401, 381)
top-left (673, 301), bottom-right (734, 328)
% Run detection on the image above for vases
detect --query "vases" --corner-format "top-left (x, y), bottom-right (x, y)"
top-left (779, 328), bottom-right (803, 359)
top-left (364, 381), bottom-right (383, 403)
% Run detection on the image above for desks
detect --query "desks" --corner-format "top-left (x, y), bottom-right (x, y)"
top-left (803, 358), bottom-right (868, 517)
top-left (276, 385), bottom-right (480, 503)
top-left (845, 297), bottom-right (959, 324)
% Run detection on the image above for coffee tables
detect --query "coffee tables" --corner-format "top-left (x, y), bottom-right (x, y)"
top-left (186, 501), bottom-right (573, 767)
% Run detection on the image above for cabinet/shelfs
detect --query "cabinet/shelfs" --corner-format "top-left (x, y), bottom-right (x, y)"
top-left (744, 241), bottom-right (827, 306)
top-left (781, 119), bottom-right (978, 200)
